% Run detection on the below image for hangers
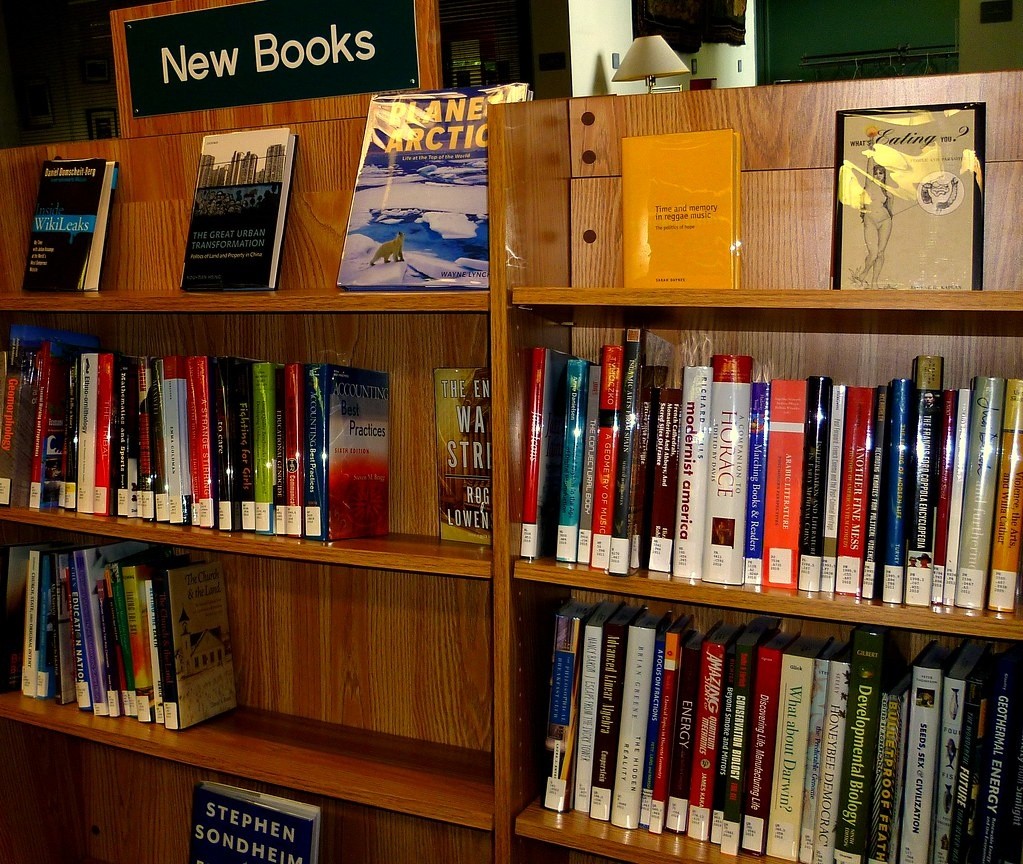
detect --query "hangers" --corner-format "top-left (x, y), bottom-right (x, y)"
top-left (814, 52), bottom-right (940, 82)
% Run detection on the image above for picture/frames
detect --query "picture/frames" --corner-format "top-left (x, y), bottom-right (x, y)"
top-left (738, 60), bottom-right (742, 72)
top-left (828, 101), bottom-right (986, 294)
top-left (980, 1), bottom-right (1013, 24)
top-left (612, 53), bottom-right (619, 69)
top-left (691, 59), bottom-right (697, 75)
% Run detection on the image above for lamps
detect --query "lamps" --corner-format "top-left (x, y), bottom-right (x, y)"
top-left (611, 35), bottom-right (691, 94)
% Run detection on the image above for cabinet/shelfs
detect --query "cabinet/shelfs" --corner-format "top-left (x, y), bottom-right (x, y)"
top-left (2, 69), bottom-right (1023, 864)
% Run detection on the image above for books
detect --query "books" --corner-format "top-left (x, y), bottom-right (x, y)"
top-left (3, 324), bottom-right (389, 731)
top-left (192, 777), bottom-right (322, 864)
top-left (180, 128), bottom-right (299, 290)
top-left (435, 366), bottom-right (497, 544)
top-left (830, 101), bottom-right (987, 291)
top-left (622, 130), bottom-right (742, 289)
top-left (337, 83), bottom-right (534, 284)
top-left (22, 157), bottom-right (118, 291)
top-left (521, 326), bottom-right (1021, 863)
